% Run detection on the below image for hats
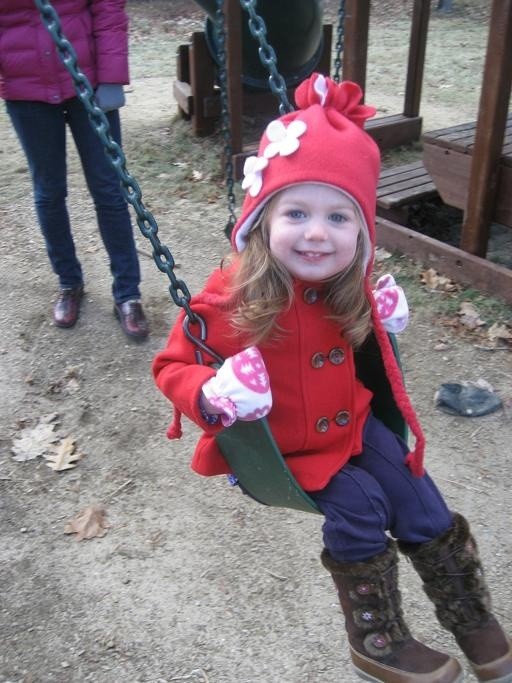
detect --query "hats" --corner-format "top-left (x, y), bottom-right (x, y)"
top-left (231, 73), bottom-right (381, 277)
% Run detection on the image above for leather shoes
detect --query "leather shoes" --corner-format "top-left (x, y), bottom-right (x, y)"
top-left (53, 286), bottom-right (84, 328)
top-left (113, 300), bottom-right (149, 338)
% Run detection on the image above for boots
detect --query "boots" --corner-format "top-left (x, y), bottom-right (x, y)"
top-left (398, 514), bottom-right (512, 683)
top-left (321, 537), bottom-right (464, 683)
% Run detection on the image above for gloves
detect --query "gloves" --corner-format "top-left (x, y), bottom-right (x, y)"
top-left (201, 346), bottom-right (272, 427)
top-left (371, 274), bottom-right (409, 333)
top-left (95, 84), bottom-right (125, 113)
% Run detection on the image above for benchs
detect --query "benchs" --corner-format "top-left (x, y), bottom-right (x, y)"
top-left (225, 108), bottom-right (422, 186)
top-left (366, 156), bottom-right (440, 225)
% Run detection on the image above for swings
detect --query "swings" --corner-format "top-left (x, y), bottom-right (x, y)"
top-left (214, 1), bottom-right (348, 251)
top-left (31, 2), bottom-right (405, 515)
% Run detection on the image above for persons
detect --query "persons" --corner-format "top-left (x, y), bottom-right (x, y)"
top-left (152, 71), bottom-right (512, 683)
top-left (0, 0), bottom-right (150, 340)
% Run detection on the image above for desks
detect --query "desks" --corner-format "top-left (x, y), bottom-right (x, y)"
top-left (419, 116), bottom-right (512, 228)
